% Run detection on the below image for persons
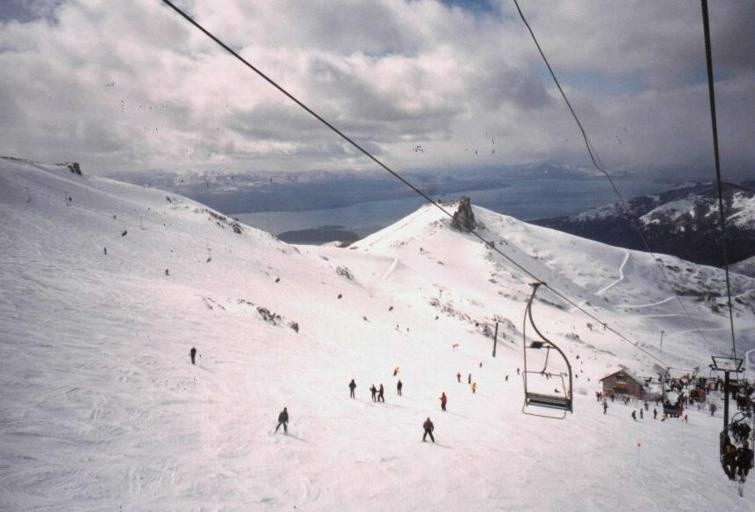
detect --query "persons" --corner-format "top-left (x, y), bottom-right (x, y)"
top-left (370, 384), bottom-right (377, 402)
top-left (190, 346), bottom-right (196, 364)
top-left (471, 382), bottom-right (477, 394)
top-left (423, 416), bottom-right (434, 443)
top-left (276, 407), bottom-right (288, 432)
top-left (480, 362), bottom-right (482, 368)
top-left (468, 373), bottom-right (471, 384)
top-left (593, 372), bottom-right (725, 422)
top-left (722, 437), bottom-right (736, 481)
top-left (393, 366), bottom-right (400, 376)
top-left (517, 368), bottom-right (520, 374)
top-left (377, 384), bottom-right (384, 402)
top-left (541, 371), bottom-right (551, 378)
top-left (439, 392), bottom-right (447, 411)
top-left (349, 379), bottom-right (356, 398)
top-left (397, 380), bottom-right (402, 396)
top-left (457, 372), bottom-right (461, 383)
top-left (736, 441), bottom-right (753, 482)
top-left (505, 375), bottom-right (509, 381)
top-left (555, 389), bottom-right (559, 393)
